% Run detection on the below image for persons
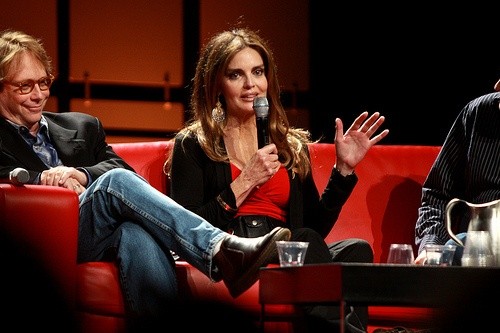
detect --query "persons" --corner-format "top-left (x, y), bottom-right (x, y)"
top-left (164, 28), bottom-right (390, 333)
top-left (0, 31), bottom-right (291, 333)
top-left (415, 81), bottom-right (500, 263)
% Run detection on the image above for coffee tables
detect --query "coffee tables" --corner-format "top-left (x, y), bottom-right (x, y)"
top-left (258, 261), bottom-right (500, 333)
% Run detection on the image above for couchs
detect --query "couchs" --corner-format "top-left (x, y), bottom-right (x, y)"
top-left (0, 142), bottom-right (453, 333)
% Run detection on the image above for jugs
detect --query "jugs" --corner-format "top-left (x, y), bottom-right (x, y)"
top-left (444, 198), bottom-right (500, 268)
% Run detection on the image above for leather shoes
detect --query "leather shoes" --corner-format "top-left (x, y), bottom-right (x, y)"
top-left (210, 226), bottom-right (291, 299)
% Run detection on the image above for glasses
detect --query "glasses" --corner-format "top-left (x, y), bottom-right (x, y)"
top-left (0, 73), bottom-right (55, 95)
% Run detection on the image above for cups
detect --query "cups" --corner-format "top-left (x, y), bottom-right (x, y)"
top-left (387, 243), bottom-right (414, 265)
top-left (275, 240), bottom-right (309, 268)
top-left (424, 244), bottom-right (457, 266)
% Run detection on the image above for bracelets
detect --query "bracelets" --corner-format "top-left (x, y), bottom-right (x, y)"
top-left (217, 194), bottom-right (238, 213)
